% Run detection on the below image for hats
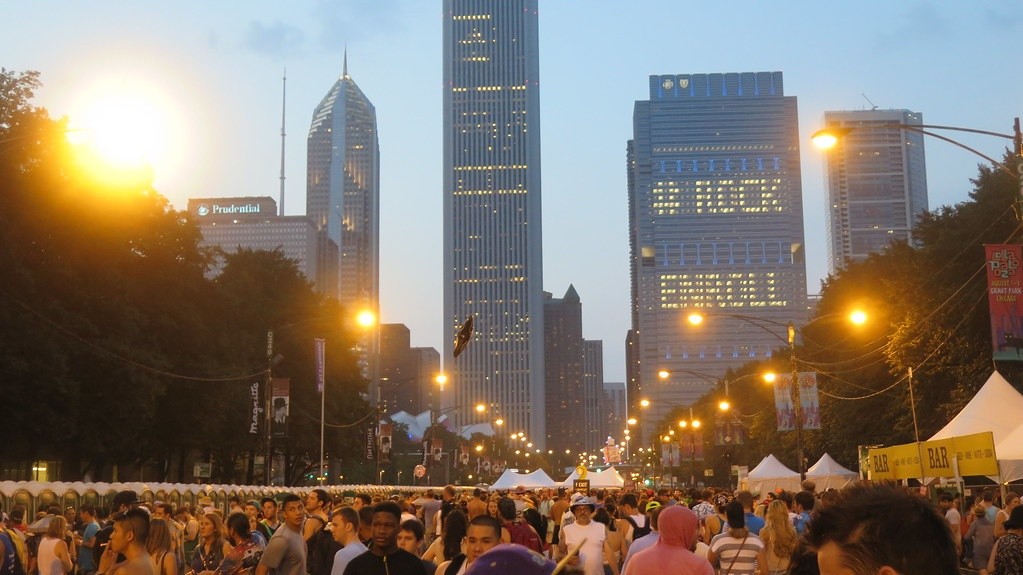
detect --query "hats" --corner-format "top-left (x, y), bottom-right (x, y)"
top-left (971, 504), bottom-right (987, 515)
top-left (9, 510), bottom-right (24, 524)
top-left (646, 501), bottom-right (661, 511)
top-left (262, 496), bottom-right (277, 506)
top-left (570, 496), bottom-right (595, 513)
top-left (726, 501), bottom-right (744, 528)
top-left (199, 496), bottom-right (211, 506)
top-left (112, 490), bottom-right (146, 504)
top-left (462, 543), bottom-right (584, 575)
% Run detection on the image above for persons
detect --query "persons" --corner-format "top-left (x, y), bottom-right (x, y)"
top-left (265, 397), bottom-right (290, 437)
top-left (458, 454), bottom-right (469, 469)
top-left (0, 483), bottom-right (1023, 575)
top-left (376, 436), bottom-right (393, 463)
top-left (483, 462), bottom-right (489, 470)
top-left (494, 465), bottom-right (499, 473)
top-left (500, 465), bottom-right (504, 472)
top-left (434, 448), bottom-right (441, 461)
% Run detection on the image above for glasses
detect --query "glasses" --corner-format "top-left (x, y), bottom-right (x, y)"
top-left (122, 510), bottom-right (135, 533)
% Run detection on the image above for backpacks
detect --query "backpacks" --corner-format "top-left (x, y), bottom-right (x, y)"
top-left (625, 516), bottom-right (652, 541)
top-left (500, 519), bottom-right (543, 555)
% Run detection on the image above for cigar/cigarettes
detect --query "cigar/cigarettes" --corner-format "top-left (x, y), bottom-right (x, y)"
top-left (100, 543), bottom-right (108, 546)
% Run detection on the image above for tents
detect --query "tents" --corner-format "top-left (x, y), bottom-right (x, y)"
top-left (902, 368), bottom-right (1023, 506)
top-left (489, 468), bottom-right (626, 489)
top-left (804, 451), bottom-right (858, 494)
top-left (738, 454), bottom-right (801, 499)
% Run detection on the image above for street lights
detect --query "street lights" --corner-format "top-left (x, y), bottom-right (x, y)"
top-left (375, 371), bottom-right (448, 485)
top-left (263, 309), bottom-right (375, 488)
top-left (430, 401), bottom-right (487, 471)
top-left (690, 308), bottom-right (867, 484)
top-left (660, 368), bottom-right (778, 470)
top-left (641, 398), bottom-right (731, 484)
